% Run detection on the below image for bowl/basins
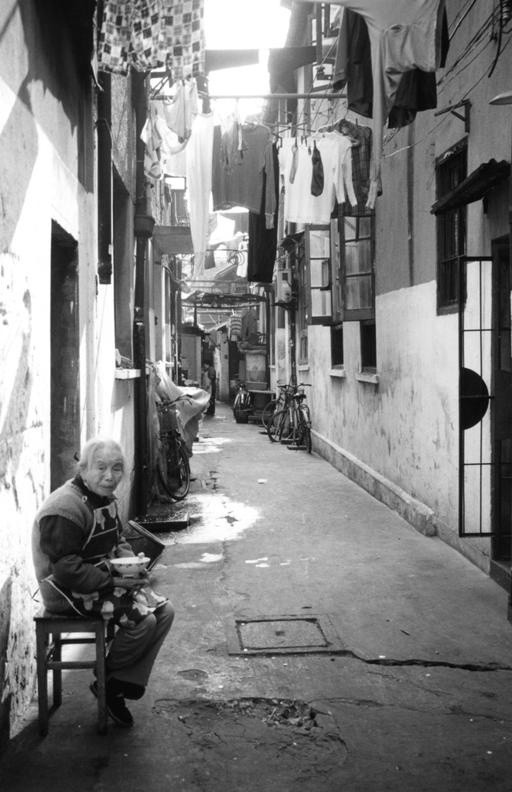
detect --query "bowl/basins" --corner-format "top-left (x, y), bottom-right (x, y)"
top-left (110, 557), bottom-right (151, 577)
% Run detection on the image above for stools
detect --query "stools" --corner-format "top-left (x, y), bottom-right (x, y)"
top-left (33, 609), bottom-right (112, 732)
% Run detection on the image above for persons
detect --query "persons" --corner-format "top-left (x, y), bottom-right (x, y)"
top-left (32, 436), bottom-right (176, 728)
top-left (201, 358), bottom-right (217, 416)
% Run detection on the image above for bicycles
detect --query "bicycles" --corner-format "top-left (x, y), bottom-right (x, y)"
top-left (262, 378), bottom-right (313, 454)
top-left (233, 382), bottom-right (251, 410)
top-left (152, 393), bottom-right (198, 501)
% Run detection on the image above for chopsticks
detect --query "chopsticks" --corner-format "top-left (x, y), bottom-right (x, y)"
top-left (127, 559), bottom-right (159, 597)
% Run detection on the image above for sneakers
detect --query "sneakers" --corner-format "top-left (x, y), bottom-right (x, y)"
top-left (91, 668), bottom-right (146, 728)
